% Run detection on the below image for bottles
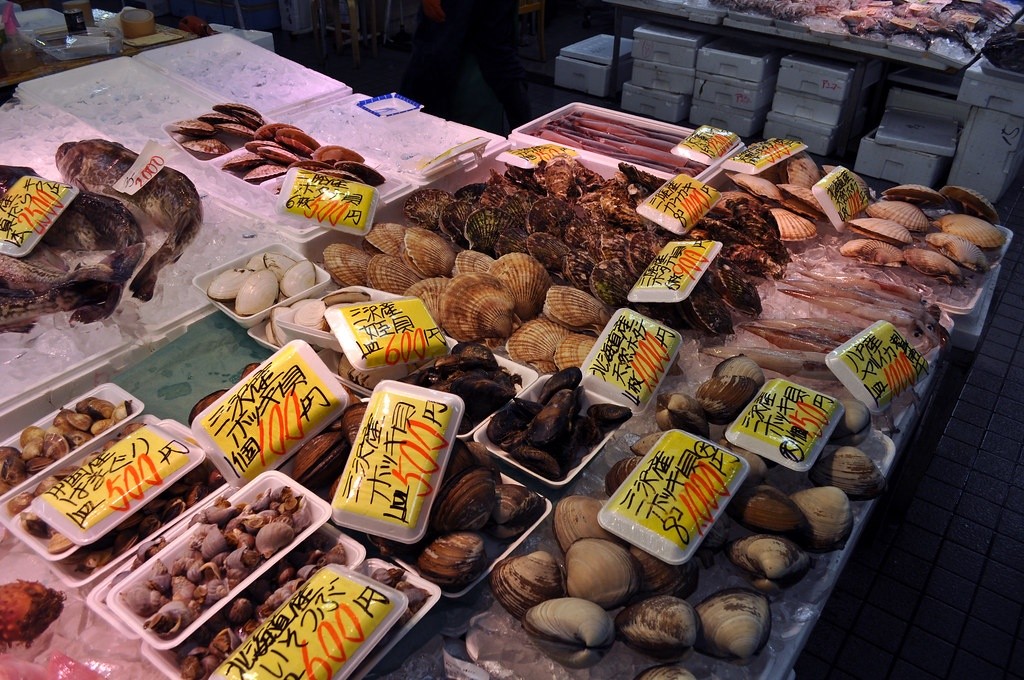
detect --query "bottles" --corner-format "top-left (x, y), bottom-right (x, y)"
top-left (63, 9), bottom-right (88, 36)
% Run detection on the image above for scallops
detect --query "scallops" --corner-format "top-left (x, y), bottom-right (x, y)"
top-left (207, 149), bottom-right (1005, 380)
top-left (172, 101), bottom-right (385, 202)
top-left (0, 341), bottom-right (891, 680)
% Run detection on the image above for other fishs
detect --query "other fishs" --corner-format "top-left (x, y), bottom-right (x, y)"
top-left (0, 140), bottom-right (204, 334)
